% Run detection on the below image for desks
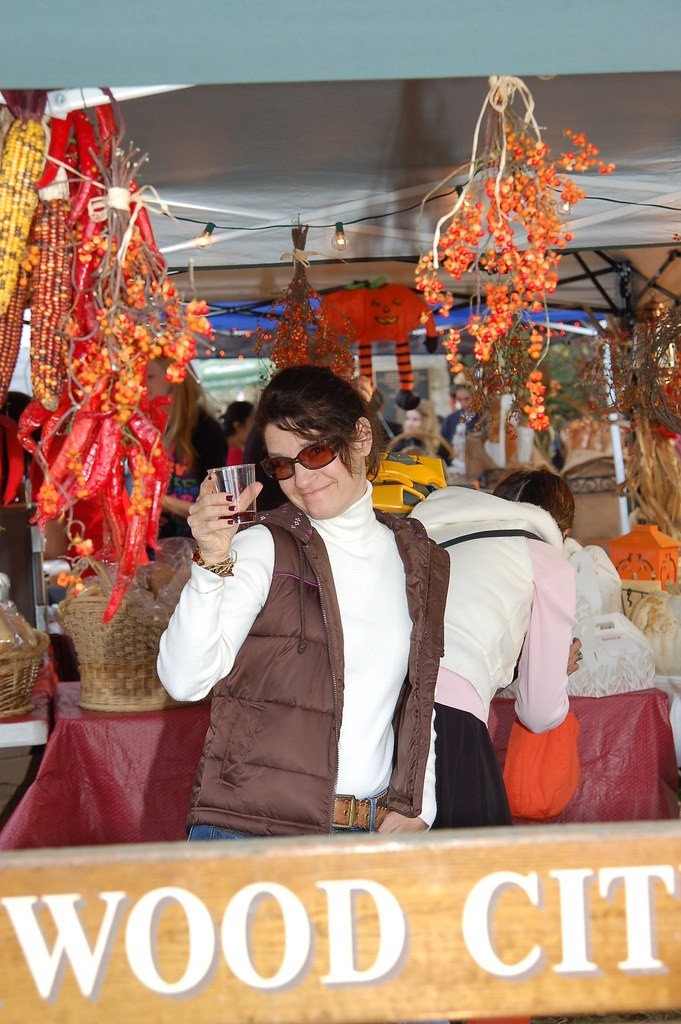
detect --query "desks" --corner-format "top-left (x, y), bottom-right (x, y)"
top-left (0, 699), bottom-right (49, 827)
top-left (0, 682), bottom-right (681, 859)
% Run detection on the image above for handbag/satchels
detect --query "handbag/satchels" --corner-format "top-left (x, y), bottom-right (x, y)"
top-left (502, 711), bottom-right (581, 820)
top-left (465, 437), bottom-right (497, 473)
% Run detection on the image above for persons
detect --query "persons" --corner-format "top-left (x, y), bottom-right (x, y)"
top-left (0, 351), bottom-right (491, 580)
top-left (407, 470), bottom-right (583, 830)
top-left (157, 366), bottom-right (452, 841)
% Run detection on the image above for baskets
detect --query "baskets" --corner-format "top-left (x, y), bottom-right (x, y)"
top-left (0, 603), bottom-right (51, 718)
top-left (56, 556), bottom-right (214, 712)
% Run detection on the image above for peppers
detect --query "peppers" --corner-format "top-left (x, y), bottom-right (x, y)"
top-left (17, 102), bottom-right (171, 623)
top-left (0, 416), bottom-right (26, 505)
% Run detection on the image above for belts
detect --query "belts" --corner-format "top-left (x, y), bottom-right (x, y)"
top-left (332, 793), bottom-right (392, 830)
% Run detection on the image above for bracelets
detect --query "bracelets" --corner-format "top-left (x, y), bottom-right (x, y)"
top-left (190, 546), bottom-right (238, 577)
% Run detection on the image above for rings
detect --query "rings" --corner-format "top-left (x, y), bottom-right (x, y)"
top-left (576, 652), bottom-right (583, 661)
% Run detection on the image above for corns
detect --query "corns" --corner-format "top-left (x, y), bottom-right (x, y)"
top-left (0, 116), bottom-right (73, 412)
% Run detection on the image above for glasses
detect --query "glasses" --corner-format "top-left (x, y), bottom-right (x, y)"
top-left (260, 437), bottom-right (341, 480)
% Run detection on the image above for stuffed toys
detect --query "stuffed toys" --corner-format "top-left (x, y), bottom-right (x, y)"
top-left (318, 276), bottom-right (440, 410)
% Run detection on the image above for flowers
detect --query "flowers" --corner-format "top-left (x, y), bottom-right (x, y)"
top-left (576, 300), bottom-right (681, 437)
top-left (254, 247), bottom-right (362, 391)
top-left (458, 315), bottom-right (541, 441)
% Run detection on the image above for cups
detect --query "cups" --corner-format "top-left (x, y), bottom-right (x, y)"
top-left (207, 464), bottom-right (257, 524)
